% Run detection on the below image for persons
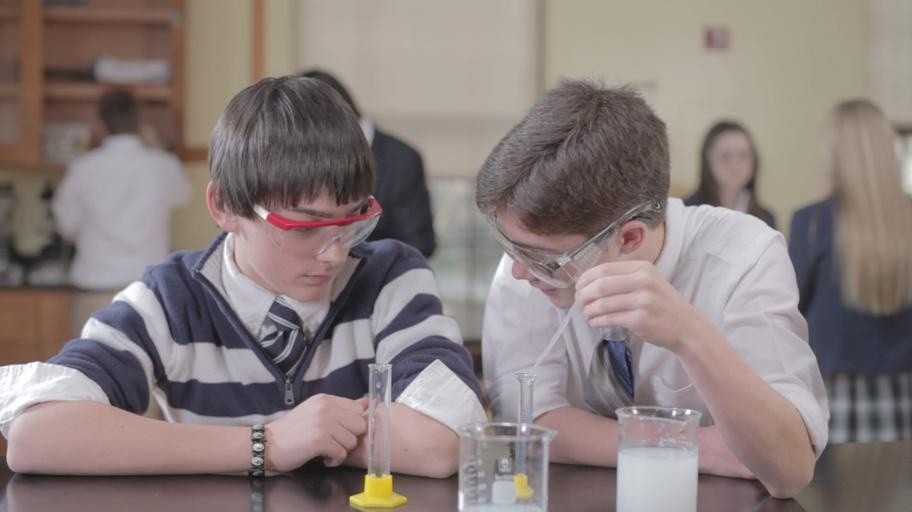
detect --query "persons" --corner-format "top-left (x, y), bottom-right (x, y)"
top-left (475, 78), bottom-right (830, 499)
top-left (683, 121), bottom-right (777, 230)
top-left (788, 99), bottom-right (912, 447)
top-left (51, 90), bottom-right (193, 292)
top-left (299, 69), bottom-right (437, 260)
top-left (0, 75), bottom-right (488, 478)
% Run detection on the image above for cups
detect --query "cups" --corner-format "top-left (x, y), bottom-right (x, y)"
top-left (614, 405), bottom-right (700, 511)
top-left (456, 420), bottom-right (559, 512)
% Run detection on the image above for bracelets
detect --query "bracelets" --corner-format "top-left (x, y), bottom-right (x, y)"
top-left (249, 424), bottom-right (266, 479)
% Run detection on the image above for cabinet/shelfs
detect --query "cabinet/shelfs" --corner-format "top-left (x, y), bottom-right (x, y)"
top-left (0, 0), bottom-right (187, 366)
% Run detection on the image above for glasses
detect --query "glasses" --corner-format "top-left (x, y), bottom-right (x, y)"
top-left (488, 200), bottom-right (660, 290)
top-left (250, 203), bottom-right (382, 257)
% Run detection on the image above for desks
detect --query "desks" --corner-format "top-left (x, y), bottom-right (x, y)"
top-left (0, 443), bottom-right (912, 512)
